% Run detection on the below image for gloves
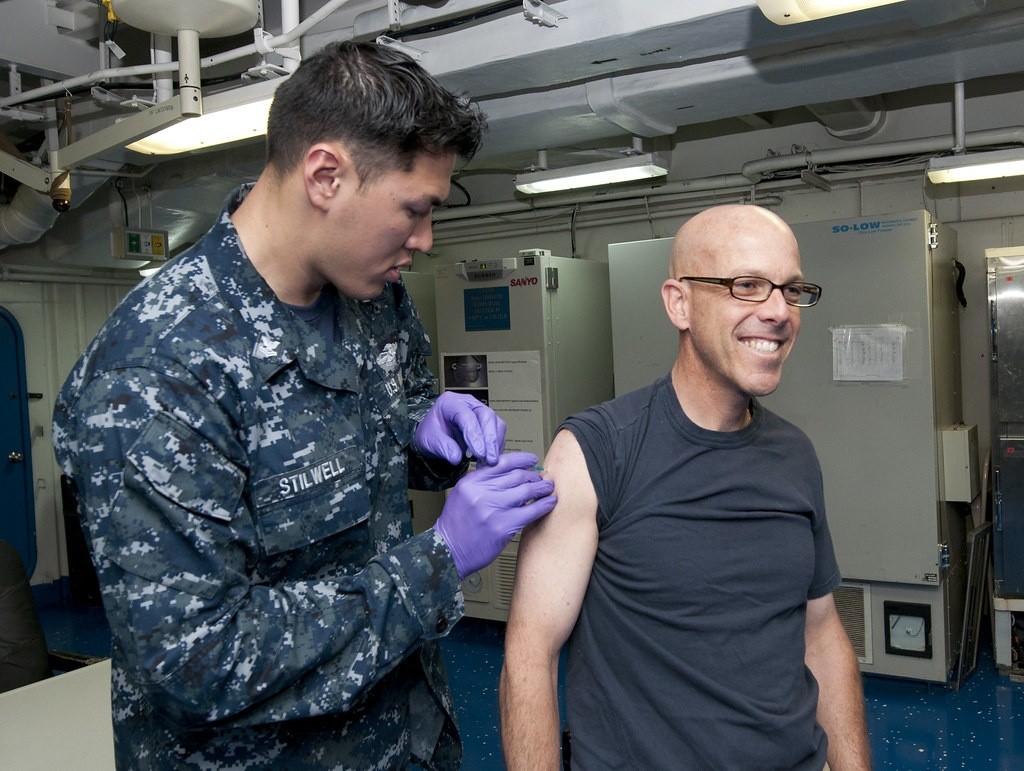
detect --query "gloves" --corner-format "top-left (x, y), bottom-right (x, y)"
top-left (433, 452), bottom-right (558, 580)
top-left (414, 392), bottom-right (506, 466)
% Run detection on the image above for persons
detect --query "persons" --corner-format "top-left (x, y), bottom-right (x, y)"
top-left (496, 201), bottom-right (874, 771)
top-left (52, 42), bottom-right (559, 771)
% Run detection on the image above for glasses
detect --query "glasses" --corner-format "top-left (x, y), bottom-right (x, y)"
top-left (679, 273), bottom-right (822, 307)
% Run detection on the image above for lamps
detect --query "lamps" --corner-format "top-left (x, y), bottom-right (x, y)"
top-left (928, 147), bottom-right (1023, 182)
top-left (513, 151), bottom-right (669, 193)
top-left (115, 75), bottom-right (290, 156)
